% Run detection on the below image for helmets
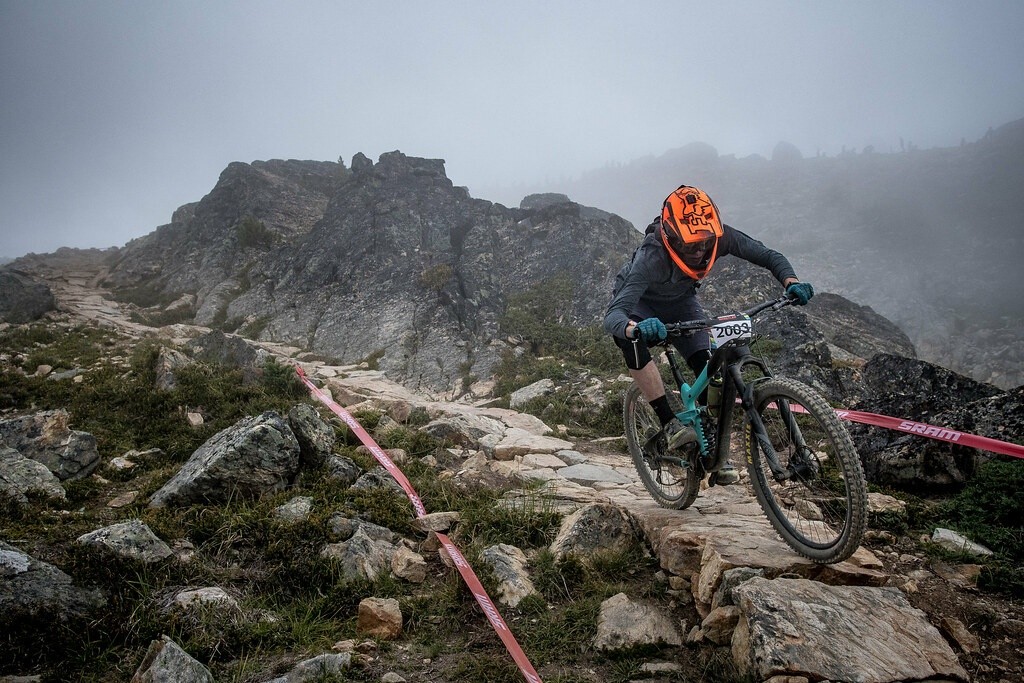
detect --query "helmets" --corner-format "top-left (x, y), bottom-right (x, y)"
top-left (661, 185), bottom-right (724, 280)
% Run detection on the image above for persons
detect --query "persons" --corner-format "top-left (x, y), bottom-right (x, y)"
top-left (605, 186), bottom-right (814, 486)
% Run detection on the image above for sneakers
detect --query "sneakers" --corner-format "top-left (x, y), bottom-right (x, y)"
top-left (664, 418), bottom-right (697, 452)
top-left (715, 460), bottom-right (740, 483)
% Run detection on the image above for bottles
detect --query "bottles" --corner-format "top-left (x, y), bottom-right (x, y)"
top-left (707, 371), bottom-right (724, 418)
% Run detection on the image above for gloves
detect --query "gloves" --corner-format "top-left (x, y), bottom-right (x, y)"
top-left (630, 317), bottom-right (667, 341)
top-left (785, 281), bottom-right (814, 306)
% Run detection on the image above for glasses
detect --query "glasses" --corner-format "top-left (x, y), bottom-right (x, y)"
top-left (667, 237), bottom-right (714, 254)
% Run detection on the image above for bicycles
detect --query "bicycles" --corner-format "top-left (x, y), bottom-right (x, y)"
top-left (623, 293), bottom-right (869, 563)
